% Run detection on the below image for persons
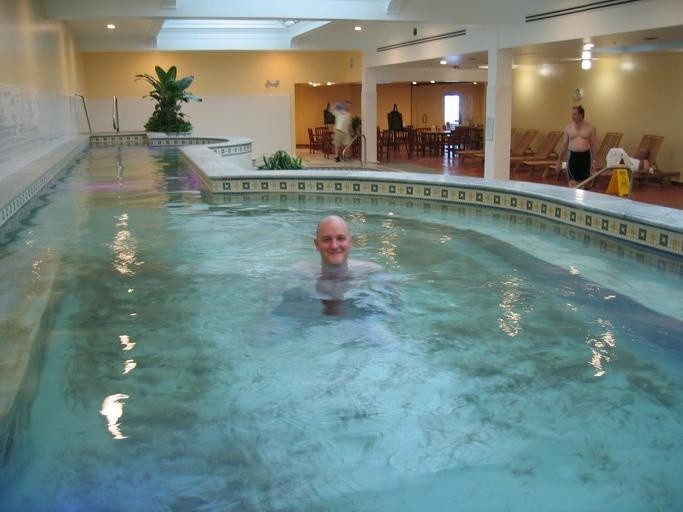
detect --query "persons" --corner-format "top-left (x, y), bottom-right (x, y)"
top-left (328, 101), bottom-right (355, 162)
top-left (262, 216), bottom-right (406, 335)
top-left (556, 106), bottom-right (599, 191)
top-left (619, 148), bottom-right (651, 175)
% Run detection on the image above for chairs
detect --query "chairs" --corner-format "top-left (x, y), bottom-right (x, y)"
top-left (541, 131), bottom-right (623, 188)
top-left (592, 134), bottom-right (679, 189)
top-left (308, 122), bottom-right (484, 167)
top-left (509, 131), bottom-right (563, 177)
